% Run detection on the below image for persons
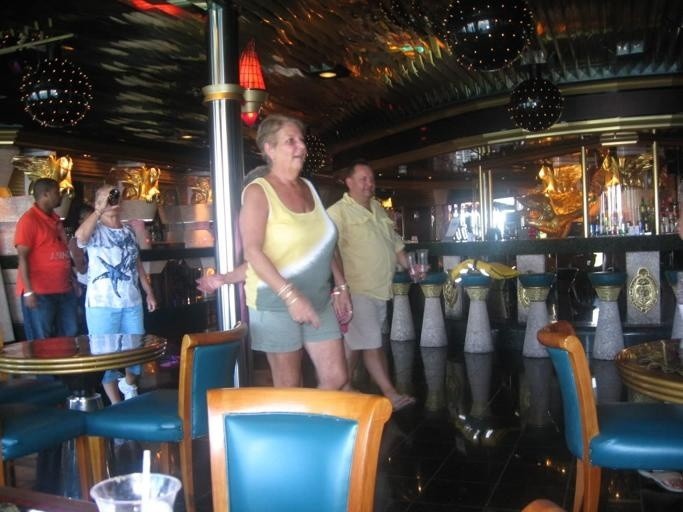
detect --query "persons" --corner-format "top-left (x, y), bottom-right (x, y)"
top-left (236, 114), bottom-right (353, 394)
top-left (10, 176), bottom-right (85, 342)
top-left (74, 205), bottom-right (94, 290)
top-left (322, 156), bottom-right (425, 414)
top-left (69, 184), bottom-right (158, 409)
top-left (191, 260), bottom-right (253, 293)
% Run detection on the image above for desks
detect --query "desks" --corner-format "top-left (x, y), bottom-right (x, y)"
top-left (0, 333), bottom-right (167, 500)
top-left (616, 339), bottom-right (683, 404)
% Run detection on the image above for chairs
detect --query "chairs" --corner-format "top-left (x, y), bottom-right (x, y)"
top-left (536, 319), bottom-right (683, 512)
top-left (0, 402), bottom-right (92, 501)
top-left (87, 321), bottom-right (247, 512)
top-left (0, 324), bottom-right (69, 406)
top-left (206, 387), bottom-right (392, 512)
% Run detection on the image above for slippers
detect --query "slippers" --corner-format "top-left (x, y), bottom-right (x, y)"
top-left (393, 395), bottom-right (415, 412)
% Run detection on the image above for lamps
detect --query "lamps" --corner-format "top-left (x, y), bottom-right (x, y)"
top-left (238, 33), bottom-right (269, 127)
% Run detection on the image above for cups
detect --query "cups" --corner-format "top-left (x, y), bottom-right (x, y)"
top-left (90, 471), bottom-right (182, 511)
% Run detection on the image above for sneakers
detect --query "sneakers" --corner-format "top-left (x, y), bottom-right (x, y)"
top-left (117, 377), bottom-right (139, 400)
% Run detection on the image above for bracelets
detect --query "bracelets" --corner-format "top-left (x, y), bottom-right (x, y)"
top-left (94, 208), bottom-right (103, 215)
top-left (224, 272), bottom-right (231, 287)
top-left (21, 290), bottom-right (34, 298)
top-left (274, 281), bottom-right (303, 308)
top-left (330, 281), bottom-right (351, 295)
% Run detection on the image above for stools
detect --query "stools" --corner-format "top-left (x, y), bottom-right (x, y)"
top-left (389, 272), bottom-right (416, 341)
top-left (587, 272), bottom-right (628, 362)
top-left (516, 272), bottom-right (557, 359)
top-left (664, 268), bottom-right (683, 338)
top-left (461, 275), bottom-right (494, 354)
top-left (417, 273), bottom-right (449, 348)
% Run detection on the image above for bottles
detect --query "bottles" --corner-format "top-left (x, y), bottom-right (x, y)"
top-left (417, 249), bottom-right (429, 279)
top-left (403, 251), bottom-right (419, 284)
top-left (589, 197), bottom-right (679, 235)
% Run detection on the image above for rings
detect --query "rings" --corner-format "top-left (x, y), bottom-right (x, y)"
top-left (347, 311), bottom-right (353, 314)
